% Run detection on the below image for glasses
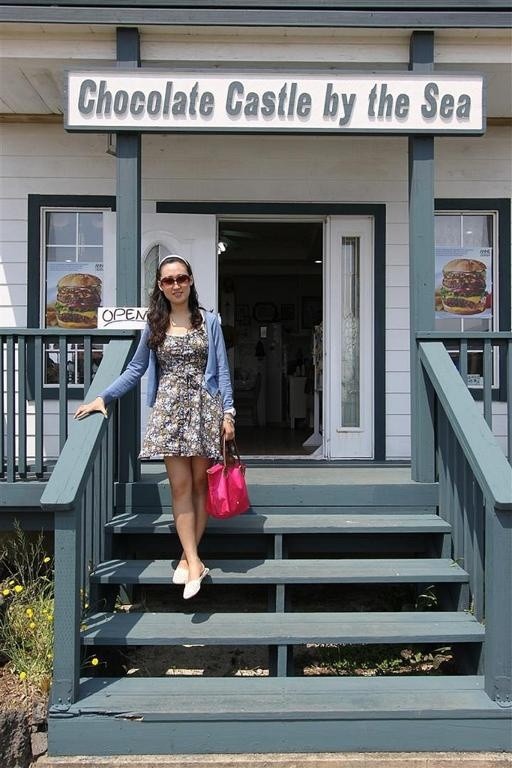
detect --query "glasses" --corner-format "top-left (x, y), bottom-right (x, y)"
top-left (158, 275), bottom-right (191, 289)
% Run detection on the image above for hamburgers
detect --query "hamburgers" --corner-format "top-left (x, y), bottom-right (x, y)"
top-left (56, 274), bottom-right (102, 328)
top-left (441, 258), bottom-right (487, 315)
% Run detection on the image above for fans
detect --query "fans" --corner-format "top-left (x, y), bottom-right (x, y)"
top-left (218, 222), bottom-right (263, 249)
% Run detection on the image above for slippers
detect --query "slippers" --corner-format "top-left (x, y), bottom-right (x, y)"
top-left (182, 568), bottom-right (209, 600)
top-left (172, 564), bottom-right (189, 585)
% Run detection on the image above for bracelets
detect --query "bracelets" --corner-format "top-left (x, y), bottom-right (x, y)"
top-left (224, 416), bottom-right (235, 424)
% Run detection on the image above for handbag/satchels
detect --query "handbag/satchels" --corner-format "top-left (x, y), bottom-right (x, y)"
top-left (204, 432), bottom-right (250, 519)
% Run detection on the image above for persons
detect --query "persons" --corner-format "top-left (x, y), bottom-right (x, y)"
top-left (72, 252), bottom-right (238, 601)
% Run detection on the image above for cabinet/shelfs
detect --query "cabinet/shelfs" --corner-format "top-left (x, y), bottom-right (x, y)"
top-left (288, 374), bottom-right (308, 429)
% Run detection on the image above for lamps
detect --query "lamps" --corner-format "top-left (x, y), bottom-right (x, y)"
top-left (218, 240), bottom-right (228, 255)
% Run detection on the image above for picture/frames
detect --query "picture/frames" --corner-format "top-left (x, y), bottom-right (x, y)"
top-left (281, 303), bottom-right (295, 320)
top-left (301, 295), bottom-right (322, 329)
top-left (235, 304), bottom-right (250, 321)
top-left (253, 303), bottom-right (278, 323)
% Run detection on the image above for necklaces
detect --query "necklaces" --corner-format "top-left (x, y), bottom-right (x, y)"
top-left (169, 313), bottom-right (189, 325)
